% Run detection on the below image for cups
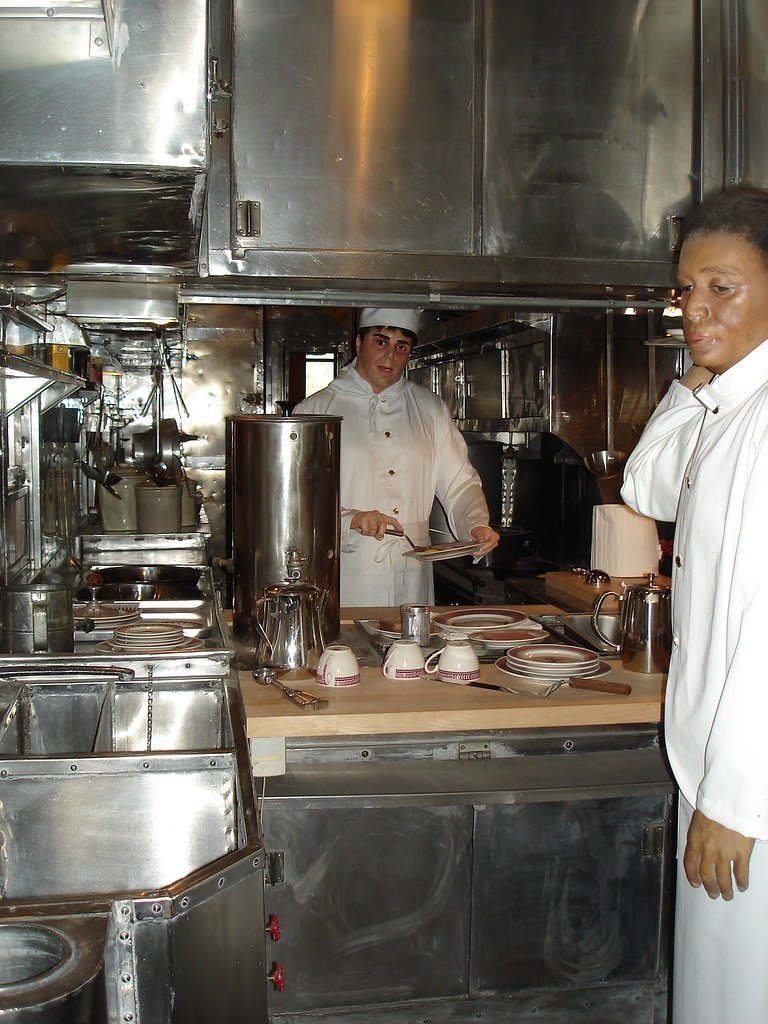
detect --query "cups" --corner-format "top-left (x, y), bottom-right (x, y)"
top-left (316, 645), bottom-right (361, 688)
top-left (401, 604), bottom-right (430, 646)
top-left (382, 640), bottom-right (426, 680)
top-left (424, 640), bottom-right (482, 682)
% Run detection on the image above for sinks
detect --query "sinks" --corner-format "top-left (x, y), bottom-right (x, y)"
top-left (93, 678), bottom-right (236, 753)
top-left (0, 684), bottom-right (110, 756)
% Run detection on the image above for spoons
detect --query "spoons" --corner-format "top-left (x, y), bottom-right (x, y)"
top-left (252, 667), bottom-right (310, 709)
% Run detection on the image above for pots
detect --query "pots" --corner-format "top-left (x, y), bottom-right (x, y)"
top-left (93, 567), bottom-right (202, 600)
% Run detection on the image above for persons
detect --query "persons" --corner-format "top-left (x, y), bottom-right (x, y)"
top-left (292, 308), bottom-right (500, 607)
top-left (620, 185), bottom-right (768, 1024)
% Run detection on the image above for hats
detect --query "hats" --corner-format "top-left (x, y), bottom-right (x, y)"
top-left (358, 307), bottom-right (420, 335)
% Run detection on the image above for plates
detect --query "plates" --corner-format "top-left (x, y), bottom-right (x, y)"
top-left (367, 608), bottom-right (611, 684)
top-left (73, 604), bottom-right (206, 654)
top-left (402, 540), bottom-right (489, 562)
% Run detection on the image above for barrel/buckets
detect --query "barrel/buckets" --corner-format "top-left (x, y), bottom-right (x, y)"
top-left (135, 482), bottom-right (182, 533)
top-left (95, 463), bottom-right (149, 530)
top-left (182, 478), bottom-right (198, 526)
top-left (4, 584), bottom-right (74, 654)
top-left (224, 399), bottom-right (346, 647)
top-left (74, 582), bottom-right (156, 604)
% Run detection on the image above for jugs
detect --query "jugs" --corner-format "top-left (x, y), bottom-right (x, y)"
top-left (252, 578), bottom-right (329, 670)
top-left (592, 574), bottom-right (671, 673)
